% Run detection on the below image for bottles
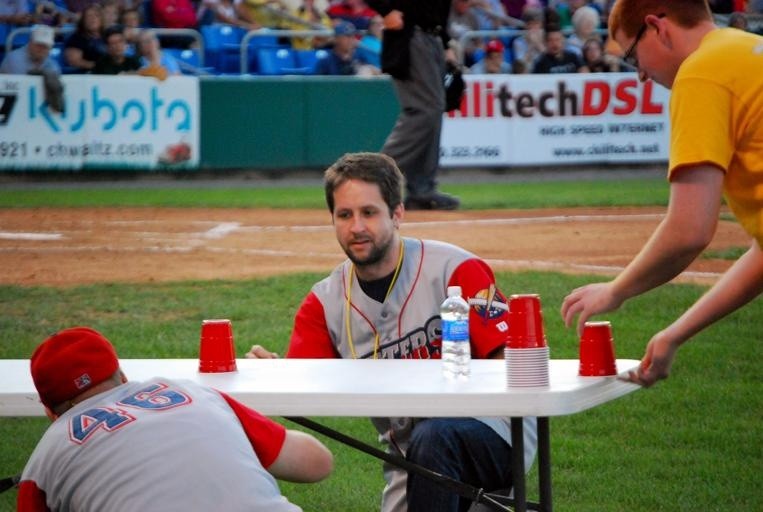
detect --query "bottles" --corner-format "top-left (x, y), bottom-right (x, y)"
top-left (437, 285), bottom-right (472, 387)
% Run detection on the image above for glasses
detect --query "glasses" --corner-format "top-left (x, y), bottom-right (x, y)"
top-left (622, 13), bottom-right (668, 69)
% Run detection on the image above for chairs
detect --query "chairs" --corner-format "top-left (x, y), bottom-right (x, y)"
top-left (1, 0), bottom-right (618, 77)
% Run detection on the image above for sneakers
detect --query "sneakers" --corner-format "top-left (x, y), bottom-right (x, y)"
top-left (405, 191), bottom-right (460, 209)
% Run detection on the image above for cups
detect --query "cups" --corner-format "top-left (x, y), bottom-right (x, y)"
top-left (577, 319), bottom-right (617, 378)
top-left (502, 293), bottom-right (550, 388)
top-left (196, 317), bottom-right (239, 374)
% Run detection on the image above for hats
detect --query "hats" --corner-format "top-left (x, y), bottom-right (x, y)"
top-left (30, 326), bottom-right (119, 407)
top-left (30, 24), bottom-right (55, 49)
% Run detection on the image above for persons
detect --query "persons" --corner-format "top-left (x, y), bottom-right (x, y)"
top-left (15, 325), bottom-right (335, 512)
top-left (1, 3), bottom-right (750, 72)
top-left (558, 0), bottom-right (762, 387)
top-left (244, 151), bottom-right (538, 511)
top-left (361, 2), bottom-right (465, 212)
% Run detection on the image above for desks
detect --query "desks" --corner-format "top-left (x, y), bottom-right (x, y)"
top-left (0, 357), bottom-right (643, 511)
